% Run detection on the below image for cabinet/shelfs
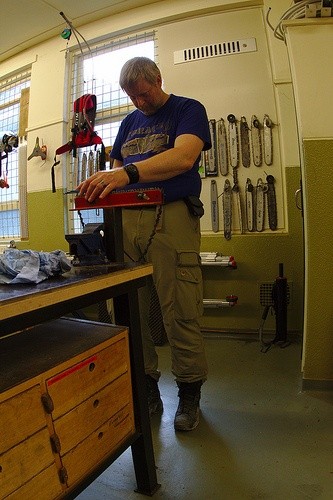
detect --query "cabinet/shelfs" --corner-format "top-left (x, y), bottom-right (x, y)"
top-left (0, 316), bottom-right (135, 499)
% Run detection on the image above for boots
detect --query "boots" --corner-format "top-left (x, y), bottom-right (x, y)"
top-left (146, 383), bottom-right (163, 415)
top-left (173, 380), bottom-right (203, 432)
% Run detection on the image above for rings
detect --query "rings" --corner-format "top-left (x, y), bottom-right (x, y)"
top-left (100, 180), bottom-right (105, 186)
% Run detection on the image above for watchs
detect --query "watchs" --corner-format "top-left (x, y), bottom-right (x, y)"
top-left (124, 163), bottom-right (138, 185)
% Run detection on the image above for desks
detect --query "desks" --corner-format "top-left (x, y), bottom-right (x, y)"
top-left (0, 263), bottom-right (160, 500)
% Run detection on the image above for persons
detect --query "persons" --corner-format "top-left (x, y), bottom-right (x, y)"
top-left (74, 56), bottom-right (214, 431)
